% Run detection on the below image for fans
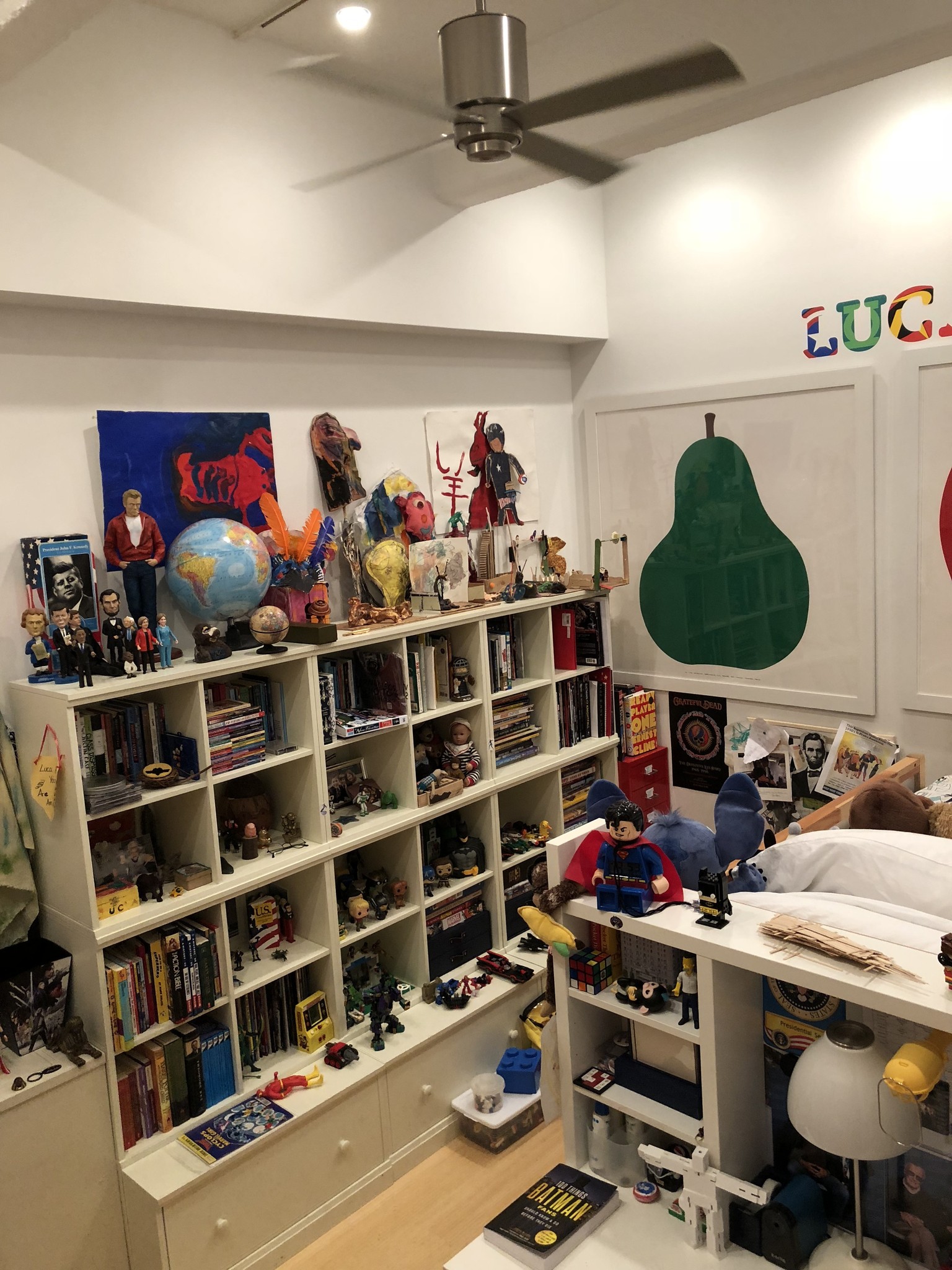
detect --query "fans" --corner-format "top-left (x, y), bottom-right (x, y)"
top-left (300, 0), bottom-right (747, 192)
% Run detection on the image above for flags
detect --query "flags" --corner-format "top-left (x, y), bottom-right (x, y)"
top-left (20, 532), bottom-right (102, 668)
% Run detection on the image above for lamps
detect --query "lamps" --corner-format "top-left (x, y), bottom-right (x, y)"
top-left (788, 1020), bottom-right (922, 1270)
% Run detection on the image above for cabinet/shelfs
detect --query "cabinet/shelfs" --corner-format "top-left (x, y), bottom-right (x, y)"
top-left (618, 745), bottom-right (671, 832)
top-left (550, 883), bottom-right (952, 1270)
top-left (6, 587), bottom-right (619, 1270)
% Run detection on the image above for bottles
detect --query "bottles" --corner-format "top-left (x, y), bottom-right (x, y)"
top-left (626, 1114), bottom-right (643, 1144)
top-left (590, 1101), bottom-right (610, 1170)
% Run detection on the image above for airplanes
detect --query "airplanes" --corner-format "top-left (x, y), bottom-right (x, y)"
top-left (517, 933), bottom-right (549, 951)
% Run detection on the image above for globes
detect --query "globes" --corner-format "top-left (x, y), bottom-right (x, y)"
top-left (250, 606), bottom-right (289, 655)
top-left (166, 518), bottom-right (271, 651)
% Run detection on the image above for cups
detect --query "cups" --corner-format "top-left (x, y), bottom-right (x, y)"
top-left (470, 1073), bottom-right (505, 1113)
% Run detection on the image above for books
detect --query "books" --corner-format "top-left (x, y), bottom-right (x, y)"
top-left (484, 1163), bottom-right (619, 1270)
top-left (76, 601), bottom-right (608, 784)
top-left (105, 917), bottom-right (310, 1163)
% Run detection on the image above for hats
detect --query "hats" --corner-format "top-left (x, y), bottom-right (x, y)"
top-left (585, 779), bottom-right (628, 822)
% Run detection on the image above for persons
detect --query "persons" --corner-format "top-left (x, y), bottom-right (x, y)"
top-left (591, 801), bottom-right (668, 917)
top-left (352, 787), bottom-right (371, 817)
top-left (186, 1040), bottom-right (201, 1057)
top-left (792, 1137), bottom-right (952, 1270)
top-left (442, 717), bottom-right (480, 787)
top-left (753, 732), bottom-right (828, 799)
top-left (448, 821), bottom-right (486, 878)
top-left (671, 954), bottom-right (700, 1030)
top-left (166, 939), bottom-right (179, 952)
top-left (345, 858), bottom-right (454, 933)
top-left (21, 490), bottom-right (176, 689)
top-left (47, 562), bottom-right (96, 620)
top-left (326, 769), bottom-right (362, 806)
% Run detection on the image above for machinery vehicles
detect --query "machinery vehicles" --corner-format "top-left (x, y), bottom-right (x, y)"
top-left (325, 1042), bottom-right (360, 1069)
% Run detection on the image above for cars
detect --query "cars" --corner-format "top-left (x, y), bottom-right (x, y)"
top-left (476, 950), bottom-right (535, 984)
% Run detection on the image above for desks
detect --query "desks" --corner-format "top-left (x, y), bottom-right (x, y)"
top-left (0, 1042), bottom-right (127, 1270)
top-left (443, 1159), bottom-right (929, 1270)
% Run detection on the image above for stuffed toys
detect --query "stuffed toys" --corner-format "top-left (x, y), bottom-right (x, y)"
top-left (612, 975), bottom-right (669, 1013)
top-left (415, 721), bottom-right (448, 800)
top-left (642, 771), bottom-right (952, 894)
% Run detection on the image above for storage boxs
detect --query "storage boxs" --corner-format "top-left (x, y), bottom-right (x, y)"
top-left (451, 1070), bottom-right (545, 1155)
top-left (586, 1120), bottom-right (664, 1189)
